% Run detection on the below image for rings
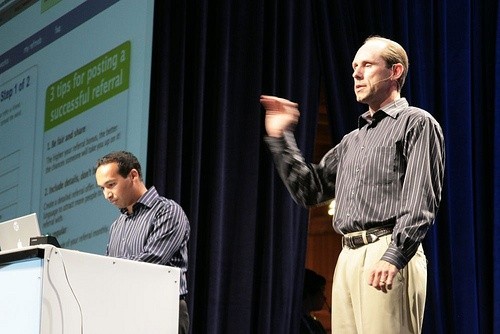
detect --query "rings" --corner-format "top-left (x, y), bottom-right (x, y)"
top-left (379, 280), bottom-right (385, 283)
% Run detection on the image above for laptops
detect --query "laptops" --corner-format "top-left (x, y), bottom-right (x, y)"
top-left (0, 213), bottom-right (42, 251)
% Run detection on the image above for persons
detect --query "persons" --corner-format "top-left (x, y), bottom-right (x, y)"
top-left (94, 151), bottom-right (191, 334)
top-left (259, 37), bottom-right (444, 334)
top-left (299, 269), bottom-right (328, 334)
